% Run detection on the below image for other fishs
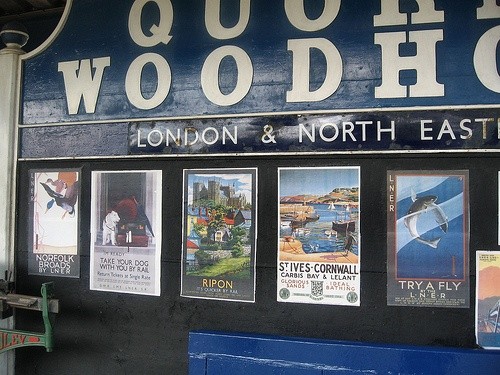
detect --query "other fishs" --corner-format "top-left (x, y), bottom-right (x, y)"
top-left (404, 188), bottom-right (448, 249)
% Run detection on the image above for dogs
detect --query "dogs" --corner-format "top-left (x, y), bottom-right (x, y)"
top-left (102, 211), bottom-right (120, 245)
